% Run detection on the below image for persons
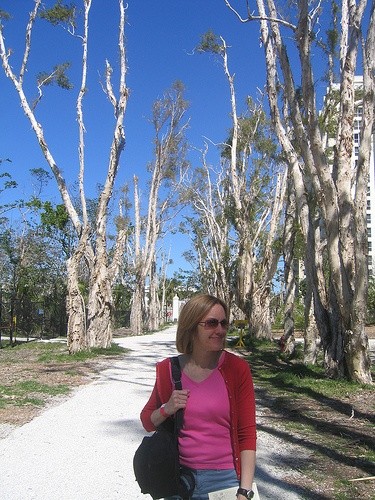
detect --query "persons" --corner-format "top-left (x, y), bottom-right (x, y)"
top-left (139, 294), bottom-right (257, 500)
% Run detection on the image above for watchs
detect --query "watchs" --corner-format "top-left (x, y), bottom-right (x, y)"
top-left (237, 488), bottom-right (255, 500)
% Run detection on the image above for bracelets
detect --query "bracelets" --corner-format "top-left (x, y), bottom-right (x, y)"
top-left (159, 403), bottom-right (172, 418)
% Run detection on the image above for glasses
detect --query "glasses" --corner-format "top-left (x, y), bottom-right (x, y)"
top-left (199, 319), bottom-right (229, 329)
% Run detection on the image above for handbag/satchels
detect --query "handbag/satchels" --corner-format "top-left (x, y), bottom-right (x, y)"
top-left (133, 357), bottom-right (185, 500)
